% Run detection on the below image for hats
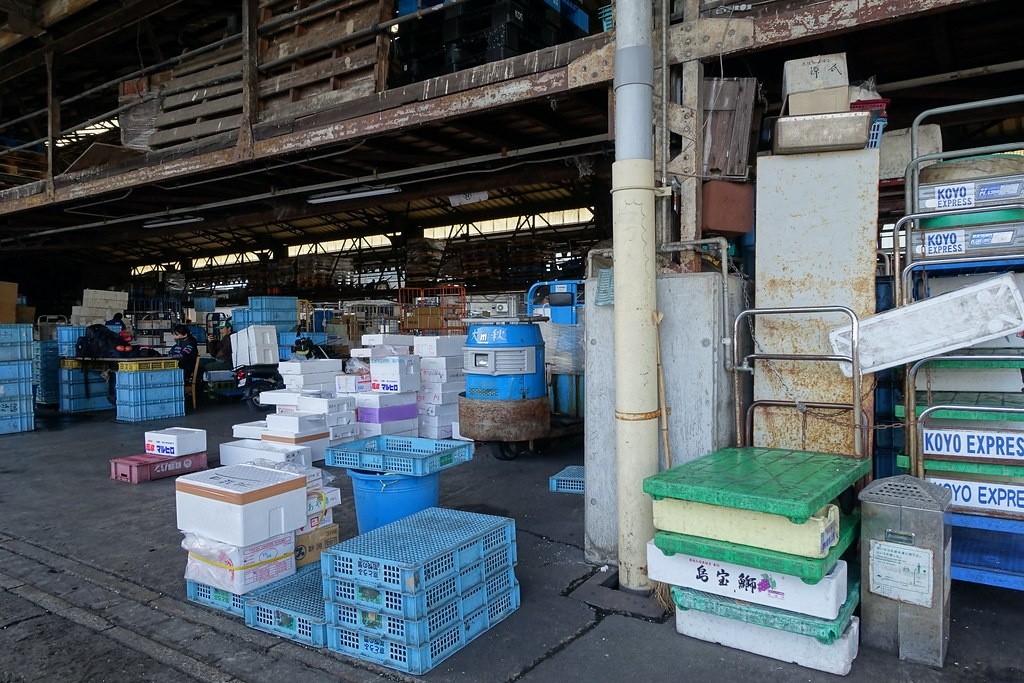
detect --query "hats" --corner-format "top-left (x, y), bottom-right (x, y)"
top-left (215, 320), bottom-right (233, 331)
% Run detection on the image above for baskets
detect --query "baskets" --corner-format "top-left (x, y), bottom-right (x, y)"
top-left (324, 435), bottom-right (476, 476)
top-left (548, 464), bottom-right (583, 493)
top-left (186, 504), bottom-right (522, 676)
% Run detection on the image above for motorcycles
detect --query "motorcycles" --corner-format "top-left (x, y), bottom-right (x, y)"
top-left (229, 324), bottom-right (326, 411)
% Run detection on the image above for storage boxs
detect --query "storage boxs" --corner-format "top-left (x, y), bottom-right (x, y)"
top-left (827, 271), bottom-right (1024, 378)
top-left (647, 498), bottom-right (860, 677)
top-left (0, 281), bottom-right (584, 675)
top-left (772, 51), bottom-right (943, 180)
top-left (702, 76), bottom-right (764, 181)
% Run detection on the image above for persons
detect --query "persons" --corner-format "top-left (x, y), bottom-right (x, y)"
top-left (196, 321), bottom-right (237, 400)
top-left (104, 312), bottom-right (126, 334)
top-left (168, 324), bottom-right (198, 414)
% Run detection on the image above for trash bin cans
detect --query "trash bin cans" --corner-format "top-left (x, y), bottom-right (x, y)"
top-left (346, 468), bottom-right (445, 534)
top-left (858, 474), bottom-right (952, 668)
